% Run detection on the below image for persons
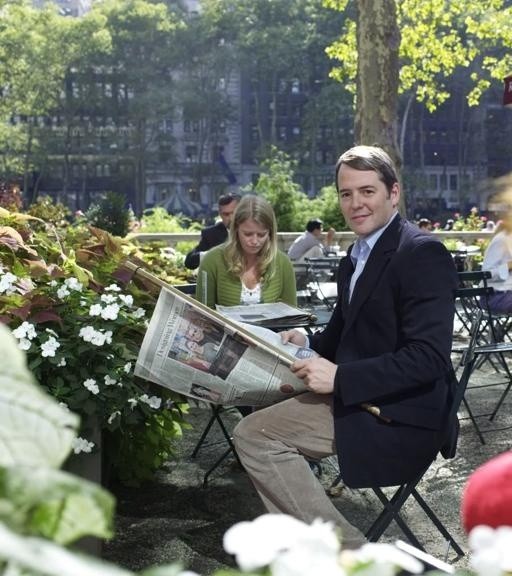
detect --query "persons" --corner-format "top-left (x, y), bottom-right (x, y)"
top-left (184, 191), bottom-right (244, 271)
top-left (173, 318), bottom-right (236, 376)
top-left (479, 215), bottom-right (512, 342)
top-left (287, 219), bottom-right (336, 261)
top-left (194, 194), bottom-right (297, 311)
top-left (192, 388), bottom-right (219, 401)
top-left (417, 217), bottom-right (431, 231)
top-left (230, 143), bottom-right (463, 550)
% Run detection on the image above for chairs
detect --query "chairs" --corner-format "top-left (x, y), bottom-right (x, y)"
top-left (170, 249), bottom-right (511, 557)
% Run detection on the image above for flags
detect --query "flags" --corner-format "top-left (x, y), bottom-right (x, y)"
top-left (218, 150), bottom-right (237, 184)
